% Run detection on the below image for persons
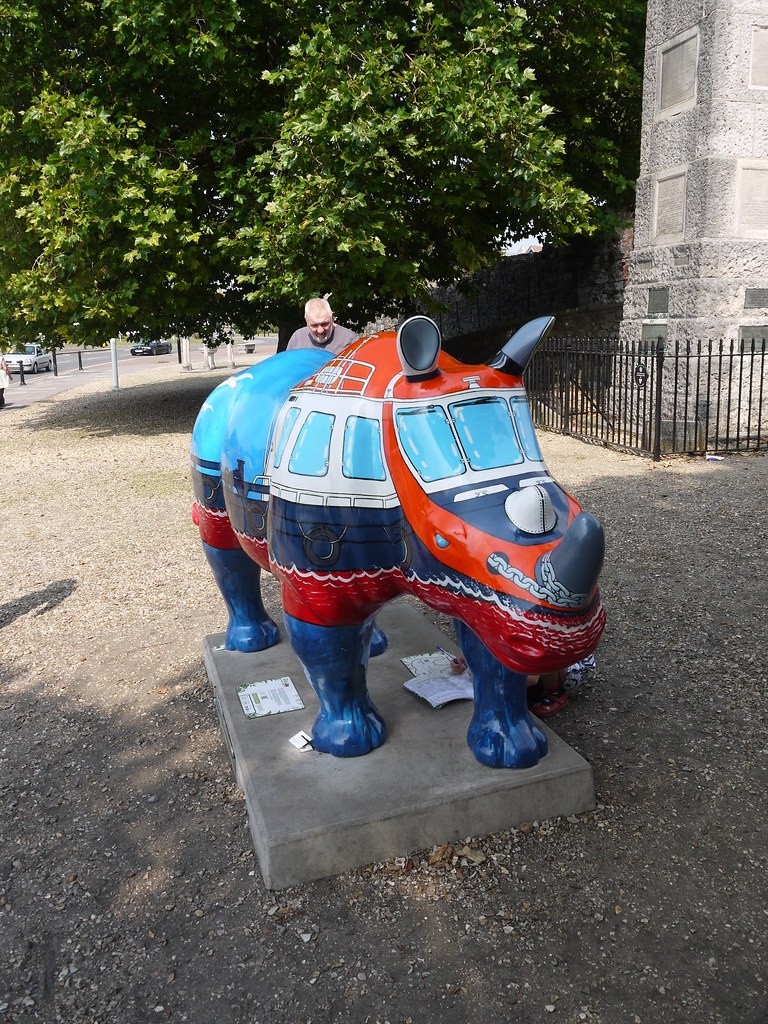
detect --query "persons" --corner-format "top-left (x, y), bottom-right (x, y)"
top-left (285, 297), bottom-right (358, 357)
top-left (0, 355), bottom-right (13, 409)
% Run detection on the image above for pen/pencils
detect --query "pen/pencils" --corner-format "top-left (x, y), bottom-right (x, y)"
top-left (436, 646), bottom-right (467, 669)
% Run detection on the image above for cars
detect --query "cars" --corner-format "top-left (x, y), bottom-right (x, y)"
top-left (130, 339), bottom-right (173, 356)
top-left (3, 343), bottom-right (53, 375)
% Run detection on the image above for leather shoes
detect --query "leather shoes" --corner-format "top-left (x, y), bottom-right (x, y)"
top-left (531, 690), bottom-right (569, 717)
